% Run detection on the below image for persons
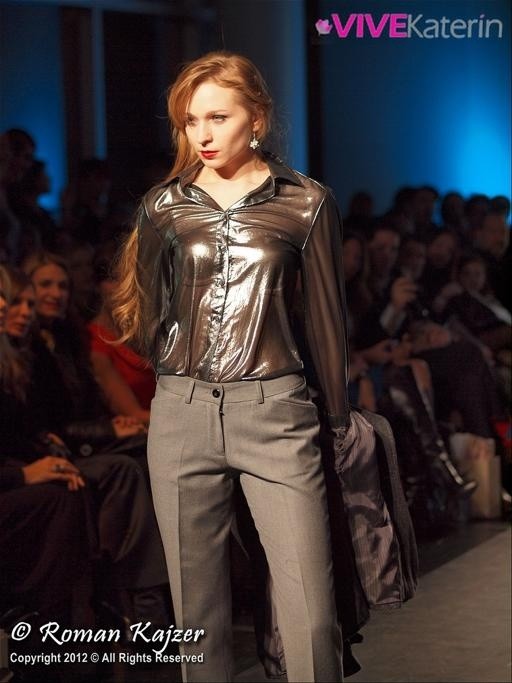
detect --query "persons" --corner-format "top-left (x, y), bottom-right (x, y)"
top-left (2, 124), bottom-right (510, 683)
top-left (113, 52), bottom-right (355, 681)
